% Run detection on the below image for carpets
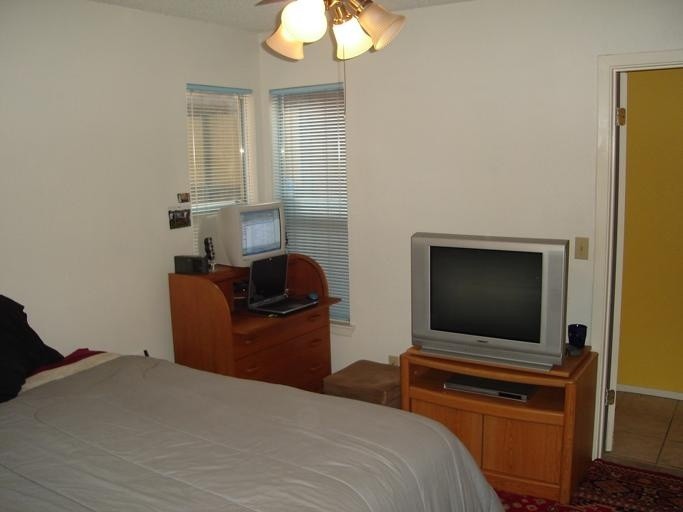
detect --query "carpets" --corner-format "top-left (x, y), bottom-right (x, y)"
top-left (496, 457), bottom-right (682, 512)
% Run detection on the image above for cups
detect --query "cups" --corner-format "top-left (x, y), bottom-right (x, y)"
top-left (569, 323), bottom-right (588, 349)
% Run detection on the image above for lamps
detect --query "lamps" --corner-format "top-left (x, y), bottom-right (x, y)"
top-left (256, 0), bottom-right (407, 62)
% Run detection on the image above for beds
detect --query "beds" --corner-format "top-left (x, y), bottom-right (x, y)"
top-left (0, 346), bottom-right (502, 510)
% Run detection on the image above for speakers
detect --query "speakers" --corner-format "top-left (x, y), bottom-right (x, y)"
top-left (567, 324), bottom-right (587, 356)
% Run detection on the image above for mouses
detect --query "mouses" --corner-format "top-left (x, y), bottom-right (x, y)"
top-left (308, 292), bottom-right (319, 300)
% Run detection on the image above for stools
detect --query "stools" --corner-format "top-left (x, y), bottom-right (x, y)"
top-left (323, 361), bottom-right (399, 405)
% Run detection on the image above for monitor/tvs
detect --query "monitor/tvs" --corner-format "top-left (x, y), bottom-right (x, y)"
top-left (199, 200), bottom-right (286, 268)
top-left (411, 233), bottom-right (569, 372)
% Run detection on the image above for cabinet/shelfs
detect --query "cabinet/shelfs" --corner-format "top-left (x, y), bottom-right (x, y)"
top-left (168, 252), bottom-right (341, 394)
top-left (400, 346), bottom-right (599, 507)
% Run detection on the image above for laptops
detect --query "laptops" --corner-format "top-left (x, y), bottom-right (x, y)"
top-left (247, 254), bottom-right (320, 316)
